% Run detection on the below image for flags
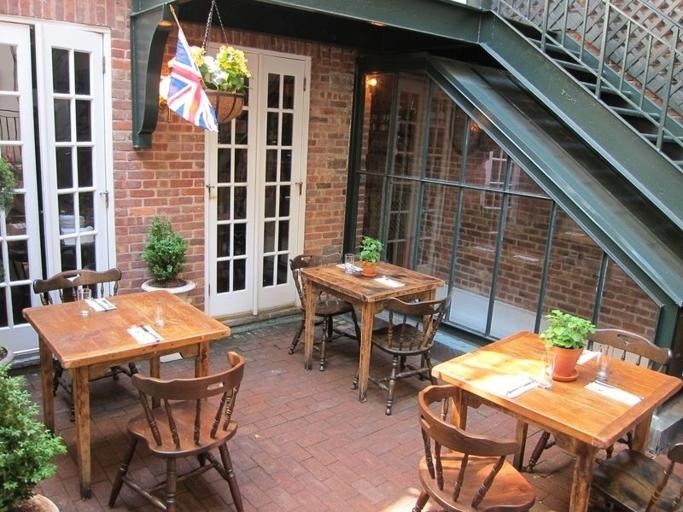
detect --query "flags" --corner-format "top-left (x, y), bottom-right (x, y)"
top-left (165, 27), bottom-right (222, 135)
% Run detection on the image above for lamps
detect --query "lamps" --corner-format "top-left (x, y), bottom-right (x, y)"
top-left (365, 78), bottom-right (376, 87)
top-left (159, 77), bottom-right (171, 110)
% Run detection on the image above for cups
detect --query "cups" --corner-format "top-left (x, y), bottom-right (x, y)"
top-left (344, 253), bottom-right (355, 276)
top-left (539, 352), bottom-right (555, 389)
top-left (153, 297), bottom-right (167, 327)
top-left (76, 288), bottom-right (92, 318)
top-left (595, 343), bottom-right (613, 381)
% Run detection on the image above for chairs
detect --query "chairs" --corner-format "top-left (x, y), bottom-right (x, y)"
top-left (289, 254), bottom-right (360, 370)
top-left (108, 354), bottom-right (246, 512)
top-left (351, 297), bottom-right (446, 416)
top-left (525, 329), bottom-right (671, 510)
top-left (590, 444), bottom-right (683, 512)
top-left (33, 269), bottom-right (138, 422)
top-left (411, 385), bottom-right (535, 512)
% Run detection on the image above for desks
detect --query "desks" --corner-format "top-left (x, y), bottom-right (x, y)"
top-left (297, 262), bottom-right (444, 403)
top-left (431, 330), bottom-right (683, 512)
top-left (22, 290), bottom-right (230, 500)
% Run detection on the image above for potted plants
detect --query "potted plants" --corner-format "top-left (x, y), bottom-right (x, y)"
top-left (141, 213), bottom-right (196, 302)
top-left (538, 311), bottom-right (594, 382)
top-left (0, 363), bottom-right (58, 512)
top-left (360, 236), bottom-right (383, 278)
top-left (0, 152), bottom-right (13, 373)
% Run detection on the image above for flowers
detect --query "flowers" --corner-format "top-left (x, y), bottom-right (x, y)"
top-left (167, 46), bottom-right (254, 93)
